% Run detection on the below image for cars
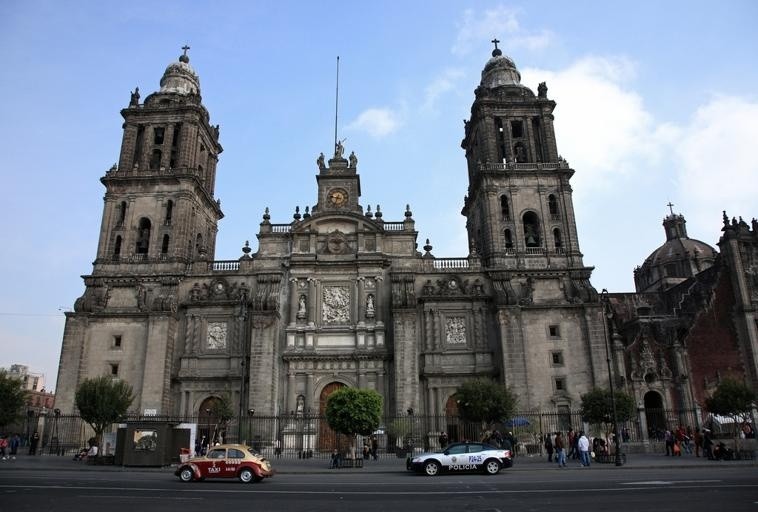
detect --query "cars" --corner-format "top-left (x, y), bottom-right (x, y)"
top-left (405, 442), bottom-right (513, 477)
top-left (173, 443), bottom-right (272, 484)
top-left (701, 413), bottom-right (752, 440)
top-left (480, 429), bottom-right (615, 456)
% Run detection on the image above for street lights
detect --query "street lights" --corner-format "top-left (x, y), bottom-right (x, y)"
top-left (205, 408), bottom-right (211, 449)
top-left (236, 292), bottom-right (250, 444)
top-left (406, 408), bottom-right (412, 457)
top-left (600, 287), bottom-right (626, 467)
top-left (53, 408), bottom-right (60, 449)
top-left (248, 408), bottom-right (254, 447)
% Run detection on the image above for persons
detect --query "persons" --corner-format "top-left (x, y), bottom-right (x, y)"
top-left (349, 152), bottom-right (357, 171)
top-left (439, 431), bottom-right (447, 449)
top-left (274, 436), bottom-right (284, 459)
top-left (538, 427), bottom-right (632, 467)
top-left (332, 448), bottom-right (339, 468)
top-left (317, 228), bottom-right (355, 253)
top-left (6, 433), bottom-right (19, 460)
top-left (0, 435), bottom-right (9, 460)
top-left (648, 421), bottom-right (758, 460)
top-left (363, 434), bottom-right (378, 460)
top-left (367, 295), bottom-right (373, 309)
top-left (299, 296), bottom-right (306, 311)
top-left (318, 153), bottom-right (328, 171)
top-left (194, 427), bottom-right (227, 457)
top-left (481, 430), bottom-right (517, 458)
top-left (335, 141), bottom-right (345, 162)
top-left (423, 430), bottom-right (430, 454)
top-left (73, 448), bottom-right (87, 462)
top-left (297, 396), bottom-right (304, 412)
top-left (28, 432), bottom-right (39, 456)
top-left (88, 444), bottom-right (98, 464)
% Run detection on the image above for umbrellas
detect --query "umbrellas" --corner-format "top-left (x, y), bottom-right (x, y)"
top-left (505, 418), bottom-right (530, 427)
